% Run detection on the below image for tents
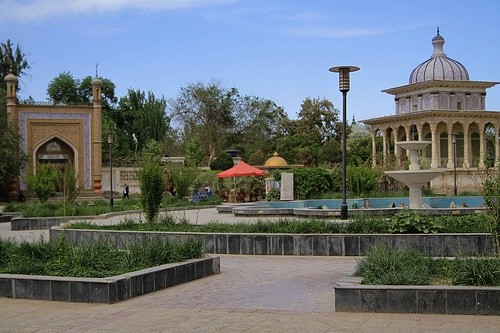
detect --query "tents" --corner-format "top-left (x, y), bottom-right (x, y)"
top-left (216, 160), bottom-right (268, 203)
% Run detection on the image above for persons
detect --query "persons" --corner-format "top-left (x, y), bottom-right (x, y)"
top-left (400, 202), bottom-right (404, 207)
top-left (388, 201), bottom-right (395, 208)
top-left (351, 203), bottom-right (358, 217)
top-left (121, 183), bottom-right (130, 201)
top-left (364, 199), bottom-right (369, 209)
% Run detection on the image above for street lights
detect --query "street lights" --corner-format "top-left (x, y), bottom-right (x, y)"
top-left (107, 131), bottom-right (114, 207)
top-left (451, 133), bottom-right (458, 196)
top-left (328, 65), bottom-right (361, 220)
